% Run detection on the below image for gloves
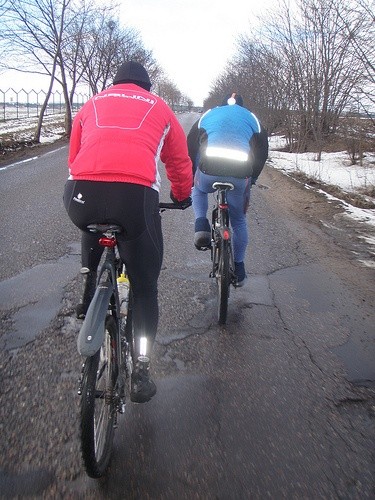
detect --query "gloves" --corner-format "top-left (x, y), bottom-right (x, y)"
top-left (170, 190), bottom-right (192, 207)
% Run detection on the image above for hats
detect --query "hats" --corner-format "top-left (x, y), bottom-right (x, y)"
top-left (112, 61), bottom-right (151, 85)
top-left (222, 92), bottom-right (243, 106)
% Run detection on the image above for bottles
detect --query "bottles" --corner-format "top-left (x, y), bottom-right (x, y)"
top-left (116, 274), bottom-right (129, 315)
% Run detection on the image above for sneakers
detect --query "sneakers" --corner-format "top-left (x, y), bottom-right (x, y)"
top-left (131, 373), bottom-right (156, 402)
top-left (234, 261), bottom-right (247, 286)
top-left (76, 302), bottom-right (91, 320)
top-left (194, 217), bottom-right (211, 246)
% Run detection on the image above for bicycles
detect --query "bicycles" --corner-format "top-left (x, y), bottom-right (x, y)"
top-left (74, 223), bottom-right (129, 478)
top-left (198, 181), bottom-right (241, 326)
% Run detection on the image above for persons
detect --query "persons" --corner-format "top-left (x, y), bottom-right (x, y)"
top-left (187, 92), bottom-right (269, 287)
top-left (62, 60), bottom-right (193, 404)
